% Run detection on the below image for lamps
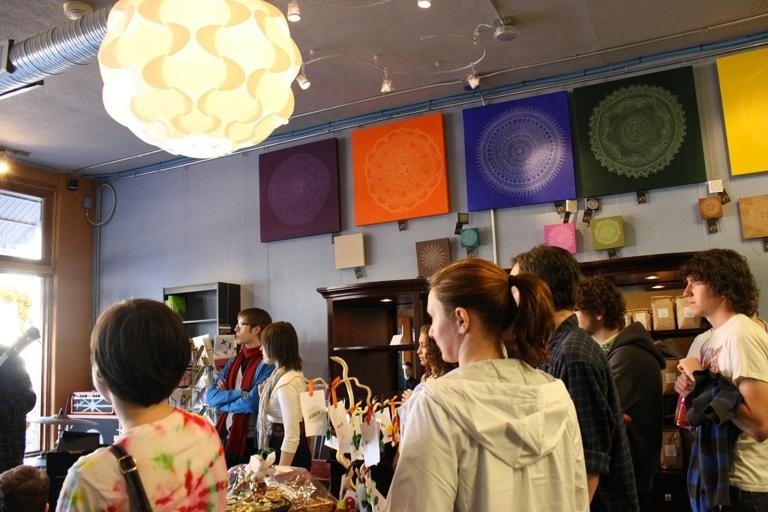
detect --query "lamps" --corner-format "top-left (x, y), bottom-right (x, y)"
top-left (96, 1), bottom-right (303, 160)
top-left (284, 0), bottom-right (433, 23)
top-left (296, 24), bottom-right (495, 89)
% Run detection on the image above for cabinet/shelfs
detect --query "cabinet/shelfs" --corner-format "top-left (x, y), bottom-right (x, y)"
top-left (162, 282), bottom-right (240, 424)
top-left (317, 248), bottom-right (713, 511)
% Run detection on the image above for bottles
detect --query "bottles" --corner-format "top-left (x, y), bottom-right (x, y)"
top-left (673, 382), bottom-right (692, 429)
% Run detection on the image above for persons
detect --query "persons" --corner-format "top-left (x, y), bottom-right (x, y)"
top-left (206, 308), bottom-right (275, 469)
top-left (398, 323), bottom-right (454, 404)
top-left (54, 298), bottom-right (228, 512)
top-left (671, 249), bottom-right (768, 511)
top-left (258, 320), bottom-right (312, 473)
top-left (510, 243), bottom-right (638, 512)
top-left (0, 465), bottom-right (50, 512)
top-left (0, 345), bottom-right (36, 476)
top-left (384, 257), bottom-right (590, 512)
top-left (572, 277), bottom-right (667, 512)
top-left (402, 361), bottom-right (418, 392)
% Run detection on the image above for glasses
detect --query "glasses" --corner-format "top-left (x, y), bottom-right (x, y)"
top-left (237, 321), bottom-right (248, 329)
